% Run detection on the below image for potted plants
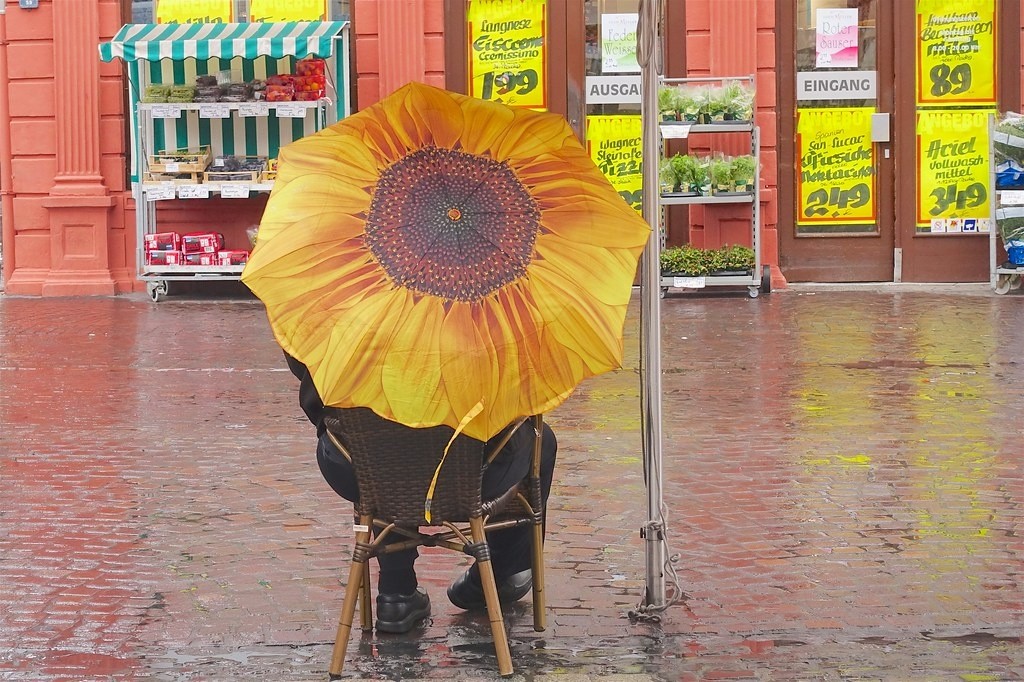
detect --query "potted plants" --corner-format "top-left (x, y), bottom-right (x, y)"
top-left (659, 240), bottom-right (756, 277)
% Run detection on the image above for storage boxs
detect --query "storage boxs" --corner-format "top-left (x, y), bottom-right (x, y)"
top-left (143, 145), bottom-right (278, 184)
top-left (142, 58), bottom-right (326, 101)
top-left (142, 231), bottom-right (250, 266)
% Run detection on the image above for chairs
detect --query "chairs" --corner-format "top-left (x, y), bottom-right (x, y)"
top-left (322, 407), bottom-right (549, 680)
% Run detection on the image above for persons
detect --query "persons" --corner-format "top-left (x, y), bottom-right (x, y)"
top-left (283, 351), bottom-right (557, 634)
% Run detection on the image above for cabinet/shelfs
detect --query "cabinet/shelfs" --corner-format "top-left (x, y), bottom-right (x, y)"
top-left (658, 74), bottom-right (772, 298)
top-left (128, 98), bottom-right (333, 303)
top-left (989, 113), bottom-right (1024, 294)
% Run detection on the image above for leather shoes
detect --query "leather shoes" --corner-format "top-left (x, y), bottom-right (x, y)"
top-left (375, 586), bottom-right (431, 633)
top-left (447, 568), bottom-right (533, 612)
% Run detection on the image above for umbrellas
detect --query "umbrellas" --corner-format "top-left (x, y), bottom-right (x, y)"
top-left (242, 81), bottom-right (648, 442)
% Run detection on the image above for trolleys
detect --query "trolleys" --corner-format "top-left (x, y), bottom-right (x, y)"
top-left (100, 20), bottom-right (350, 300)
top-left (657, 73), bottom-right (771, 297)
top-left (988, 112), bottom-right (1024, 296)
